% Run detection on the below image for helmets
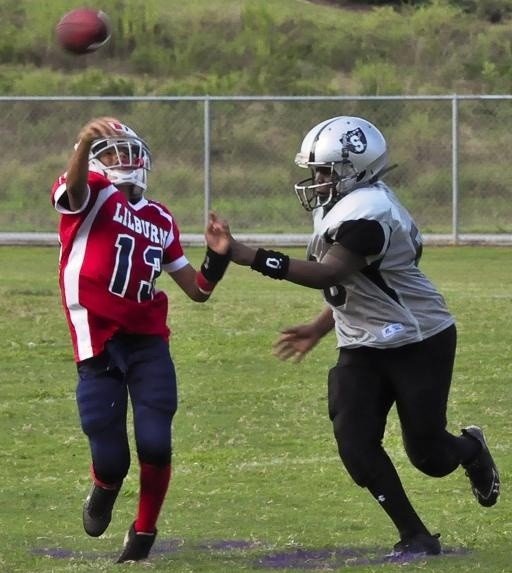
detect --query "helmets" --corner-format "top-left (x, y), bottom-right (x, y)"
top-left (74, 122), bottom-right (149, 191)
top-left (294, 115), bottom-right (389, 196)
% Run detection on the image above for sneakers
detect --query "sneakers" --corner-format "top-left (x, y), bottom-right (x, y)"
top-left (83, 480), bottom-right (123, 537)
top-left (116, 520), bottom-right (157, 563)
top-left (461, 425), bottom-right (500, 507)
top-left (384, 535), bottom-right (441, 562)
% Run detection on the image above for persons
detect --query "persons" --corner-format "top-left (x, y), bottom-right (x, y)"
top-left (47, 116), bottom-right (230, 564)
top-left (208, 113), bottom-right (500, 561)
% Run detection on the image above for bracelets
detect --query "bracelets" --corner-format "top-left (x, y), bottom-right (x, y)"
top-left (201, 245), bottom-right (234, 283)
top-left (194, 271), bottom-right (215, 295)
top-left (251, 248), bottom-right (290, 280)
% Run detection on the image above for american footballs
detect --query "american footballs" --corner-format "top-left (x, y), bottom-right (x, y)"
top-left (58, 9), bottom-right (107, 54)
